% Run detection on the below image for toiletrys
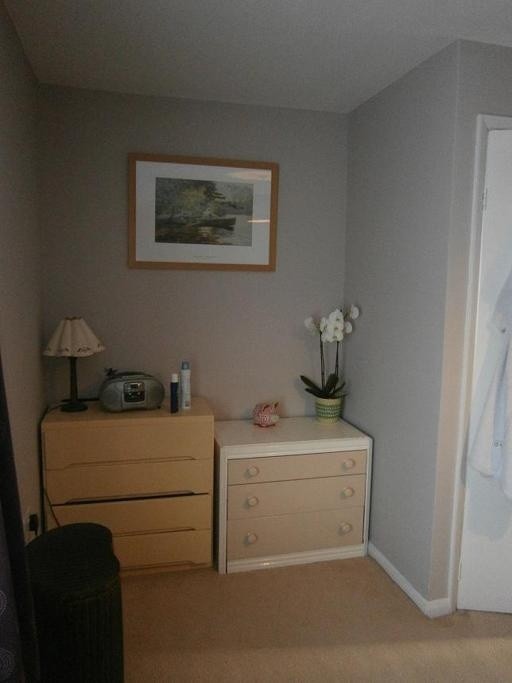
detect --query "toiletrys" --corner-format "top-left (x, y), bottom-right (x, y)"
top-left (181, 362), bottom-right (192, 411)
top-left (170, 373), bottom-right (180, 415)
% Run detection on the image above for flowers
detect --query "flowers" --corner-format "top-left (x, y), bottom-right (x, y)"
top-left (293, 304), bottom-right (362, 398)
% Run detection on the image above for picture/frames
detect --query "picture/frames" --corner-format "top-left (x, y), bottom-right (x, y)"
top-left (127, 152), bottom-right (278, 272)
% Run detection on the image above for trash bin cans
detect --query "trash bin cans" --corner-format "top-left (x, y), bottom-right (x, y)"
top-left (24, 522), bottom-right (125, 683)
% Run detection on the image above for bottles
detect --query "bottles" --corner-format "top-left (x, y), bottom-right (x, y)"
top-left (179, 360), bottom-right (192, 413)
top-left (169, 371), bottom-right (180, 415)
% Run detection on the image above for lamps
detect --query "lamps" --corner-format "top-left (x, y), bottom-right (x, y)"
top-left (41, 318), bottom-right (106, 413)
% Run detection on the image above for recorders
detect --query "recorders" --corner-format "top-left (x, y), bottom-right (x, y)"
top-left (99, 371), bottom-right (165, 413)
